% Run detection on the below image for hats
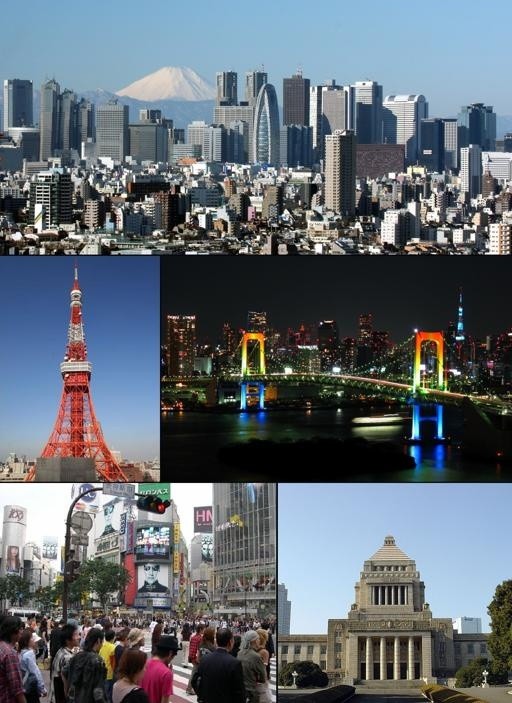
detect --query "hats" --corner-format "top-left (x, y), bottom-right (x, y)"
top-left (155, 635), bottom-right (182, 650)
top-left (127, 628), bottom-right (146, 648)
top-left (243, 631), bottom-right (259, 642)
top-left (32, 632), bottom-right (41, 643)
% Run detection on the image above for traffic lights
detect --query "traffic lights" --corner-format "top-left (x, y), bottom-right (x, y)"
top-left (137, 494), bottom-right (170, 514)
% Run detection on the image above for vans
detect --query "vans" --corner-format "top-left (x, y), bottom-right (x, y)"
top-left (7, 607), bottom-right (41, 622)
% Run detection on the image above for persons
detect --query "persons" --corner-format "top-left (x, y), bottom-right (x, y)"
top-left (7, 546), bottom-right (20, 573)
top-left (0, 610), bottom-right (276, 703)
top-left (138, 562), bottom-right (168, 592)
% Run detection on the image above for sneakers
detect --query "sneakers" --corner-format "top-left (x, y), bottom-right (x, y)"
top-left (186, 687), bottom-right (196, 695)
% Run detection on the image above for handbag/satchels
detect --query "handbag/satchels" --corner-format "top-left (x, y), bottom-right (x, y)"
top-left (23, 672), bottom-right (41, 699)
